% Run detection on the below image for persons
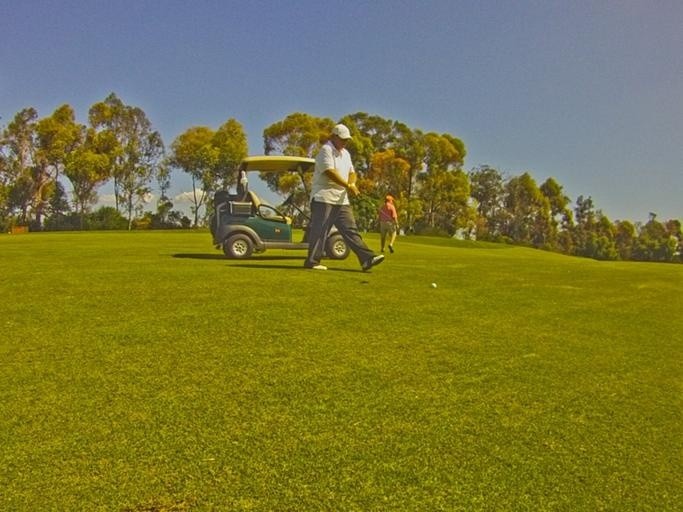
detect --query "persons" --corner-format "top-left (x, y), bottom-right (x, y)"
top-left (381, 195), bottom-right (399, 252)
top-left (304, 124), bottom-right (385, 270)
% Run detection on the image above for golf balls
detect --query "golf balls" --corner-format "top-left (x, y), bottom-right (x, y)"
top-left (430, 282), bottom-right (437, 289)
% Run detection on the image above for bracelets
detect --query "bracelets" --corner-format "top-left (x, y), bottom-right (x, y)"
top-left (344, 183), bottom-right (350, 193)
top-left (350, 183), bottom-right (355, 186)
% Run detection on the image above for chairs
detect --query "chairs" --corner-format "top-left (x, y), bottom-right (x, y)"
top-left (250, 188), bottom-right (292, 225)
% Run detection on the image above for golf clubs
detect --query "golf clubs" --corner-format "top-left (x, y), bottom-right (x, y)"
top-left (360, 194), bottom-right (400, 234)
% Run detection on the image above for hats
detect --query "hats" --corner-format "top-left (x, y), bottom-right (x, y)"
top-left (385, 195), bottom-right (395, 201)
top-left (332, 123), bottom-right (353, 140)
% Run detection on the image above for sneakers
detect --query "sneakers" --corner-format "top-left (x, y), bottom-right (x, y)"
top-left (381, 244), bottom-right (394, 253)
top-left (311, 264), bottom-right (328, 270)
top-left (361, 255), bottom-right (385, 272)
